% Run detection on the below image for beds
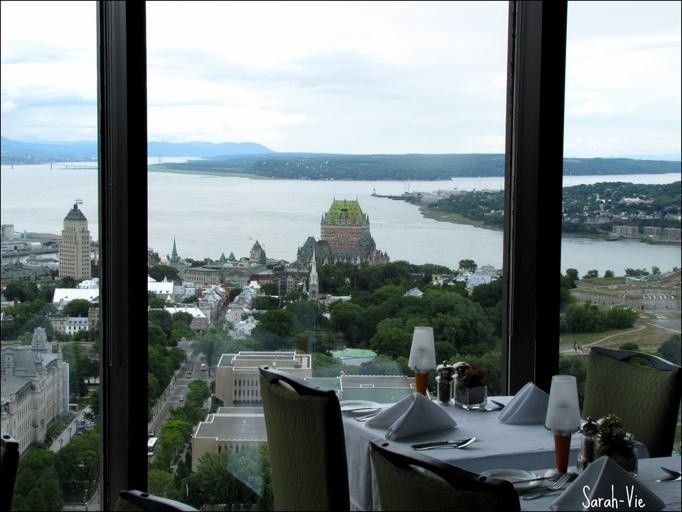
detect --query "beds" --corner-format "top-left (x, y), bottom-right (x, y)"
top-left (338, 394), bottom-right (681, 511)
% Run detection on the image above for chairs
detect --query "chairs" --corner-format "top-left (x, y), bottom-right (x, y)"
top-left (581, 344), bottom-right (681, 457)
top-left (257, 362), bottom-right (519, 511)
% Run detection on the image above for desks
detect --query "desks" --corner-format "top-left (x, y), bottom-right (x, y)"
top-left (338, 394), bottom-right (681, 511)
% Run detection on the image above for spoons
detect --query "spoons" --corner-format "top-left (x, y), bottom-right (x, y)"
top-left (660, 466), bottom-right (682, 478)
top-left (411, 436), bottom-right (478, 451)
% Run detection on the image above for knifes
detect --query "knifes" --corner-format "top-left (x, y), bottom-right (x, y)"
top-left (412, 440), bottom-right (470, 449)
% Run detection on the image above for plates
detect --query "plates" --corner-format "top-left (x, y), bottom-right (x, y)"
top-left (479, 468), bottom-right (544, 490)
top-left (339, 400), bottom-right (381, 414)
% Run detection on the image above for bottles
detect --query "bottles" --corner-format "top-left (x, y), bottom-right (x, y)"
top-left (576, 415), bottom-right (601, 472)
top-left (435, 360), bottom-right (470, 406)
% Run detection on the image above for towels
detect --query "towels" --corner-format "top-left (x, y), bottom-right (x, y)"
top-left (497, 381), bottom-right (548, 424)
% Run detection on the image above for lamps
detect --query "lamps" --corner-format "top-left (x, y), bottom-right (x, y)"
top-left (542, 373), bottom-right (581, 482)
top-left (406, 325), bottom-right (437, 395)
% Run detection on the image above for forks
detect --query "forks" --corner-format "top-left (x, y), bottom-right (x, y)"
top-left (518, 474), bottom-right (571, 498)
